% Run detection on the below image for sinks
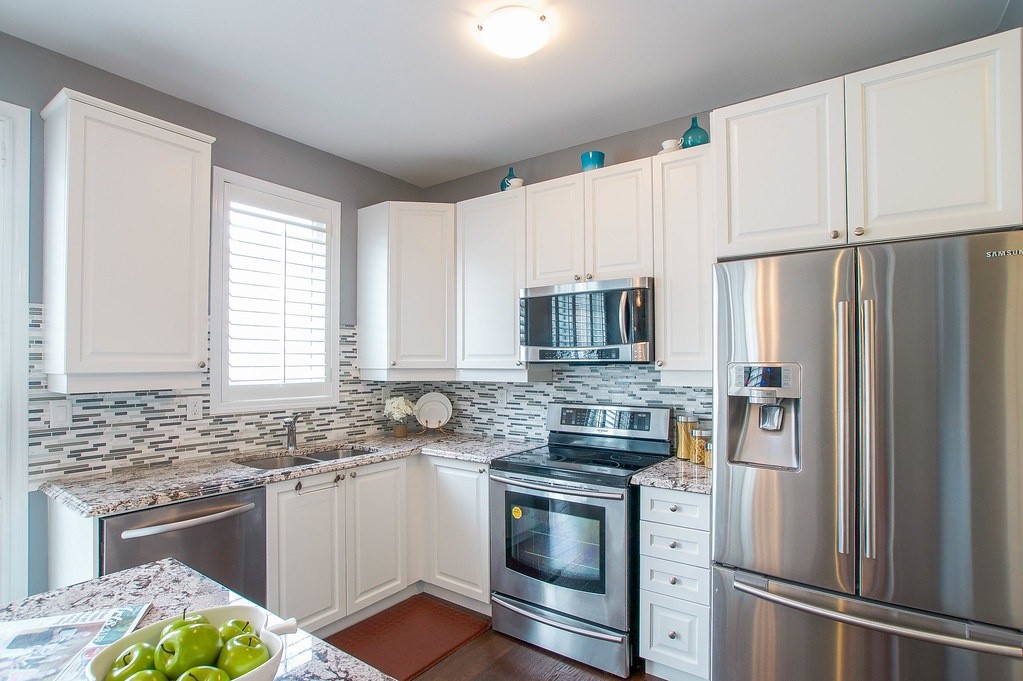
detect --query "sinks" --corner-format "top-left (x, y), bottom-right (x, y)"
top-left (230, 452), bottom-right (320, 470)
top-left (303, 446), bottom-right (375, 461)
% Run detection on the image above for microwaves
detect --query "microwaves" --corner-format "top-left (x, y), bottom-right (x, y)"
top-left (519, 277), bottom-right (655, 364)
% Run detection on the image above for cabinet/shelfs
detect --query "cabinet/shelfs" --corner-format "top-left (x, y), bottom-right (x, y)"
top-left (654, 144), bottom-right (712, 372)
top-left (41, 88), bottom-right (216, 395)
top-left (456, 187), bottom-right (526, 385)
top-left (525, 154), bottom-right (653, 287)
top-left (708, 27), bottom-right (1021, 262)
top-left (264, 458), bottom-right (408, 640)
top-left (638, 486), bottom-right (713, 681)
top-left (421, 454), bottom-right (492, 617)
top-left (383, 200), bottom-right (459, 380)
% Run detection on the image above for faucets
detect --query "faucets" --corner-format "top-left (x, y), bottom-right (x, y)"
top-left (283, 412), bottom-right (315, 451)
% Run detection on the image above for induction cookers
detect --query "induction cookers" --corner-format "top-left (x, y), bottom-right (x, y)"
top-left (491, 402), bottom-right (671, 487)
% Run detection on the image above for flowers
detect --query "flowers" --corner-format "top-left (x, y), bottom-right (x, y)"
top-left (381, 397), bottom-right (419, 422)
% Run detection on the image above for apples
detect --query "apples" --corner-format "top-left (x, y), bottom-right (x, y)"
top-left (106, 608), bottom-right (270, 681)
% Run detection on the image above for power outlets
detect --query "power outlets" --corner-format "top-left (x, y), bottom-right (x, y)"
top-left (497, 389), bottom-right (507, 407)
top-left (187, 396), bottom-right (202, 420)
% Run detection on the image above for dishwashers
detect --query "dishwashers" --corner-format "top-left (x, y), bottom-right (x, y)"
top-left (46, 485), bottom-right (268, 609)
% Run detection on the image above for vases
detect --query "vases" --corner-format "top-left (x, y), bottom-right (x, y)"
top-left (393, 424), bottom-right (407, 438)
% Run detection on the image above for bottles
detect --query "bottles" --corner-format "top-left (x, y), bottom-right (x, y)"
top-left (501, 167), bottom-right (517, 191)
top-left (676, 413), bottom-right (699, 459)
top-left (681, 116), bottom-right (709, 149)
top-left (704, 442), bottom-right (713, 469)
top-left (690, 427), bottom-right (711, 463)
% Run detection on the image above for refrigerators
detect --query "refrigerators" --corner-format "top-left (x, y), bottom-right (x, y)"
top-left (710, 230), bottom-right (1023, 681)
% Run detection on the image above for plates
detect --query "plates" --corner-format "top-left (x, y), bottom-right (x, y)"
top-left (415, 392), bottom-right (453, 429)
top-left (505, 186), bottom-right (520, 190)
top-left (657, 149), bottom-right (676, 156)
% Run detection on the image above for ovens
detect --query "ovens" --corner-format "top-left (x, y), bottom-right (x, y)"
top-left (489, 469), bottom-right (644, 679)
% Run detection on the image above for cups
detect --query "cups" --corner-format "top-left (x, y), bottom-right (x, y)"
top-left (661, 138), bottom-right (684, 149)
top-left (580, 151), bottom-right (605, 171)
top-left (504, 178), bottom-right (523, 186)
